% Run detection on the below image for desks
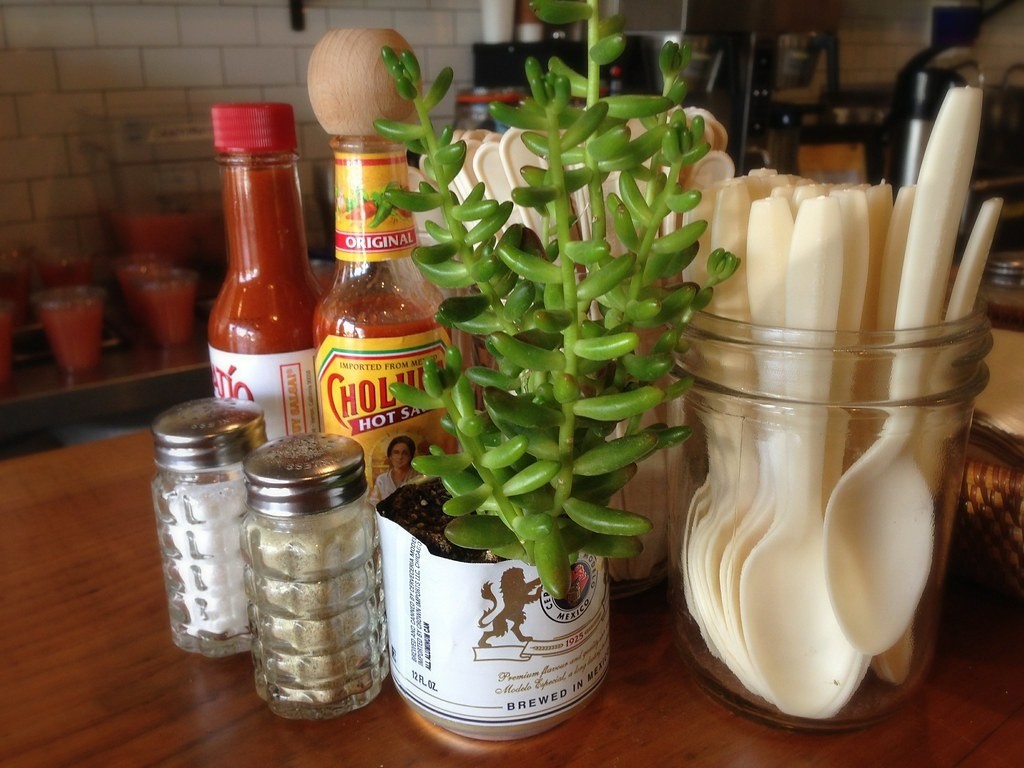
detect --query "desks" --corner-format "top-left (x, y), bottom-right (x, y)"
top-left (0, 430), bottom-right (1024, 768)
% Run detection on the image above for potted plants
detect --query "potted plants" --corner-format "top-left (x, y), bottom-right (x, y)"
top-left (366, 0), bottom-right (743, 744)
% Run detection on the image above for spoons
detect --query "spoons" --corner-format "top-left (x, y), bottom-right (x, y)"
top-left (421, 82), bottom-right (1004, 719)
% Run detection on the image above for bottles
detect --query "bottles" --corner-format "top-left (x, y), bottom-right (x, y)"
top-left (977, 251), bottom-right (1024, 336)
top-left (241, 431), bottom-right (390, 720)
top-left (311, 132), bottom-right (458, 496)
top-left (150, 396), bottom-right (270, 659)
top-left (205, 101), bottom-right (325, 441)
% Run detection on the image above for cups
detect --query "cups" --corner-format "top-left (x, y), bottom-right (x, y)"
top-left (1, 243), bottom-right (198, 383)
top-left (663, 309), bottom-right (993, 732)
